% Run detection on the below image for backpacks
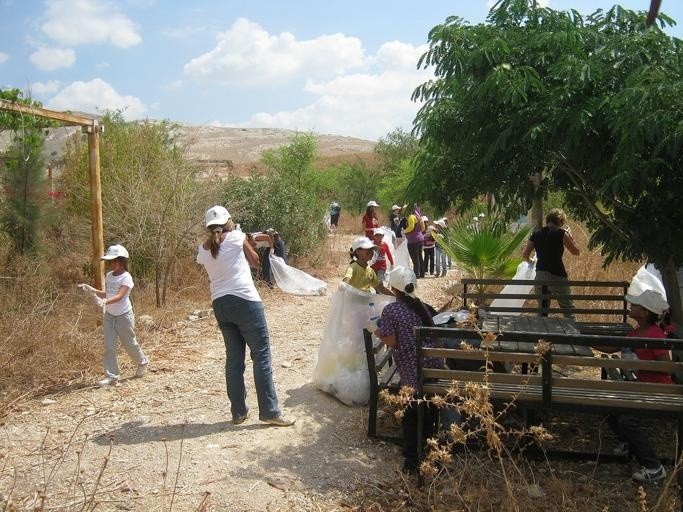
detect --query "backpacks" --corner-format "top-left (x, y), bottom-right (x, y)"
top-left (253, 227), bottom-right (281, 244)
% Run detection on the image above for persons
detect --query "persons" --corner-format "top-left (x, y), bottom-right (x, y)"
top-left (440, 217), bottom-right (452, 268)
top-left (609, 265), bottom-right (671, 481)
top-left (433, 221), bottom-right (447, 278)
top-left (362, 201), bottom-right (380, 241)
top-left (472, 213), bottom-right (485, 235)
top-left (401, 204), bottom-right (425, 279)
top-left (197, 205), bottom-right (296, 426)
top-left (390, 205), bottom-right (401, 231)
top-left (422, 216), bottom-right (434, 275)
top-left (330, 201), bottom-right (340, 226)
top-left (367, 266), bottom-right (446, 475)
top-left (371, 232), bottom-right (394, 294)
top-left (78, 244), bottom-right (149, 386)
top-left (522, 207), bottom-right (580, 320)
top-left (247, 231), bottom-right (287, 283)
top-left (344, 237), bottom-right (394, 297)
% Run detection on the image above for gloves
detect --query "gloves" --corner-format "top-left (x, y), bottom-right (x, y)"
top-left (94, 294), bottom-right (105, 308)
top-left (78, 283), bottom-right (96, 295)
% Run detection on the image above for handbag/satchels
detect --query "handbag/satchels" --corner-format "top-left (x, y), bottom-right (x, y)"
top-left (262, 228), bottom-right (279, 245)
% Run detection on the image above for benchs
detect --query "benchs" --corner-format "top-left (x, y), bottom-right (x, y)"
top-left (363, 328), bottom-right (405, 445)
top-left (460, 278), bottom-right (635, 335)
top-left (413, 326), bottom-right (683, 491)
top-left (600, 323), bottom-right (682, 462)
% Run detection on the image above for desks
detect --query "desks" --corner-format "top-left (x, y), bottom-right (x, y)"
top-left (482, 314), bottom-right (594, 454)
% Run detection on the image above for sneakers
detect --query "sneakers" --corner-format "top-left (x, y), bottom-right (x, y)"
top-left (262, 413), bottom-right (296, 426)
top-left (234, 410), bottom-right (250, 424)
top-left (614, 441), bottom-right (630, 455)
top-left (631, 464), bottom-right (667, 481)
top-left (135, 360), bottom-right (149, 377)
top-left (97, 376), bottom-right (118, 385)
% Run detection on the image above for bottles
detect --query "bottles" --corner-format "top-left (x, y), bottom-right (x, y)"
top-left (525, 263), bottom-right (534, 279)
top-left (368, 301), bottom-right (381, 329)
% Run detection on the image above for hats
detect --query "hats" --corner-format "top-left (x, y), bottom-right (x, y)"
top-left (352, 236), bottom-right (378, 252)
top-left (373, 227), bottom-right (384, 235)
top-left (100, 243), bottom-right (130, 261)
top-left (367, 201), bottom-right (380, 206)
top-left (389, 266), bottom-right (418, 299)
top-left (204, 205), bottom-right (231, 228)
top-left (625, 289), bottom-right (669, 315)
top-left (422, 216), bottom-right (428, 221)
top-left (433, 220), bottom-right (445, 228)
top-left (392, 205), bottom-right (401, 210)
